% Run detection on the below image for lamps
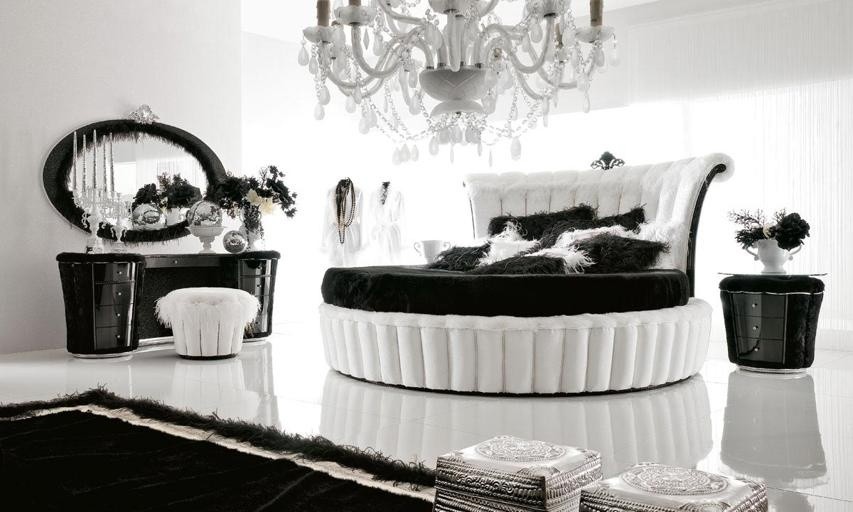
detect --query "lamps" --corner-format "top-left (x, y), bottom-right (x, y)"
top-left (295, 1), bottom-right (622, 158)
top-left (69, 130), bottom-right (131, 254)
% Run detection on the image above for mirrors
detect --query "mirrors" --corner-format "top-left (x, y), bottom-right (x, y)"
top-left (41, 120), bottom-right (229, 243)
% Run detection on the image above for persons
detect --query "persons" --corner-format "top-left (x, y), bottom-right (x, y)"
top-left (373, 179), bottom-right (402, 265)
top-left (326, 177), bottom-right (363, 267)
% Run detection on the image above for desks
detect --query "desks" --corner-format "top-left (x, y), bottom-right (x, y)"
top-left (56, 251), bottom-right (281, 357)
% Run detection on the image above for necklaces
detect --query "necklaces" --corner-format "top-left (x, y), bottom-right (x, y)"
top-left (335, 177), bottom-right (356, 246)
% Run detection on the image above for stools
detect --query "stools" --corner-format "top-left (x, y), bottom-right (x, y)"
top-left (154, 285), bottom-right (261, 358)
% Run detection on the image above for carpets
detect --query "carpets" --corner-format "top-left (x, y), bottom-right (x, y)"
top-left (0, 384), bottom-right (438, 511)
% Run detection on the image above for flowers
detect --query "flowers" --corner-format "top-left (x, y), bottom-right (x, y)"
top-left (727, 206), bottom-right (810, 251)
top-left (208, 165), bottom-right (297, 237)
top-left (156, 170), bottom-right (200, 206)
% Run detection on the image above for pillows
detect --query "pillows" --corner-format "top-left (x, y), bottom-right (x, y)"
top-left (429, 201), bottom-right (667, 276)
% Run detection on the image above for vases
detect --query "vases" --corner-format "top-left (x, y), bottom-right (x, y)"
top-left (745, 237), bottom-right (802, 273)
top-left (166, 206), bottom-right (187, 226)
top-left (238, 219), bottom-right (264, 250)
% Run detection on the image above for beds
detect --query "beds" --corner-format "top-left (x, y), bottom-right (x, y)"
top-left (320, 368), bottom-right (714, 476)
top-left (319, 150), bottom-right (734, 399)
